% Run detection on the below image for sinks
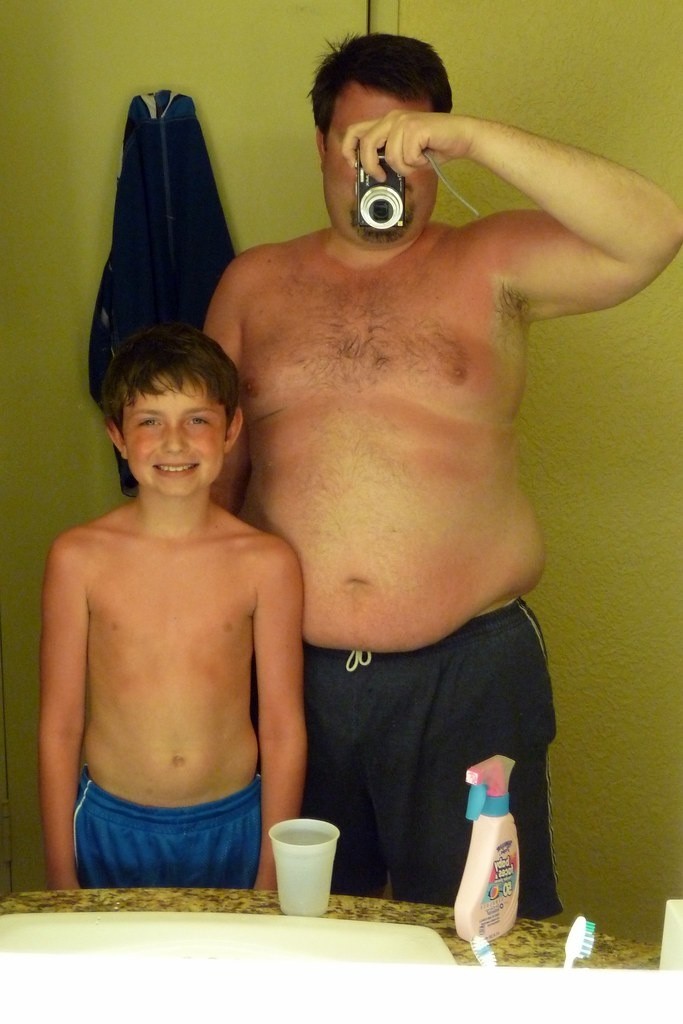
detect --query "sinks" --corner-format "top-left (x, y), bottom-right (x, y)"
top-left (0, 909), bottom-right (459, 967)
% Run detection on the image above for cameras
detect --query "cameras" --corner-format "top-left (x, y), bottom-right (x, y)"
top-left (354, 147), bottom-right (405, 229)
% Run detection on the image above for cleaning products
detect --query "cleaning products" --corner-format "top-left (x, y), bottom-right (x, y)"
top-left (453, 755), bottom-right (522, 945)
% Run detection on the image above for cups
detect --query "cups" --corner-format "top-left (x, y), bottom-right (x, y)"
top-left (266, 815), bottom-right (342, 919)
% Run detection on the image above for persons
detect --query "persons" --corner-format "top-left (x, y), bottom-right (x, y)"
top-left (203, 33), bottom-right (681, 924)
top-left (37, 325), bottom-right (307, 892)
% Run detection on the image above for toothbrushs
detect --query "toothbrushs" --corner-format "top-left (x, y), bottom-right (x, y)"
top-left (470, 931), bottom-right (500, 970)
top-left (561, 915), bottom-right (598, 969)
